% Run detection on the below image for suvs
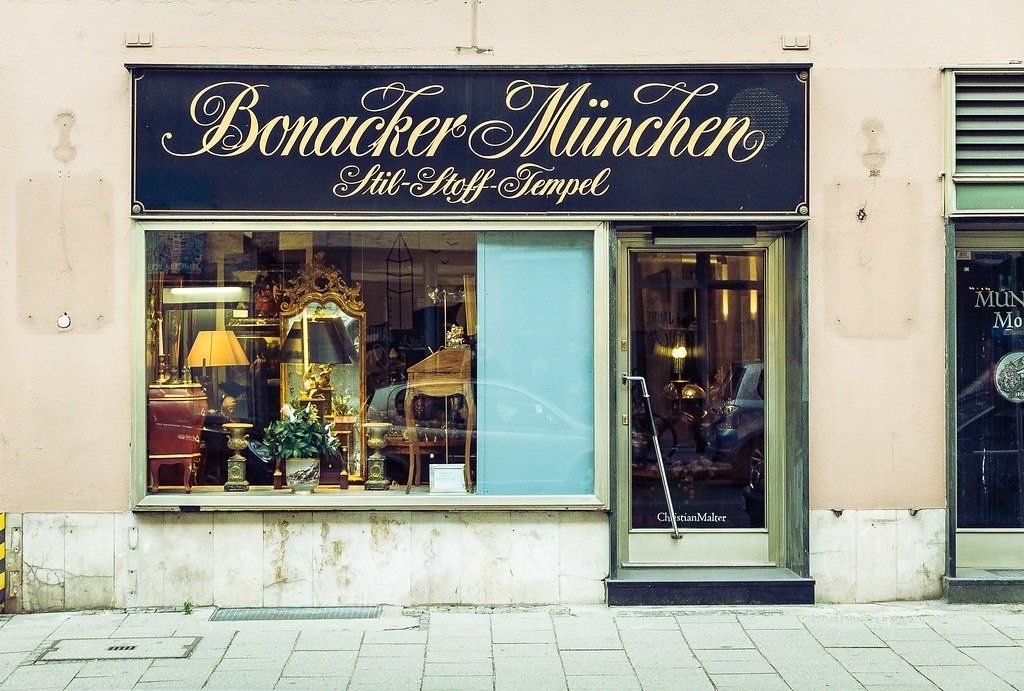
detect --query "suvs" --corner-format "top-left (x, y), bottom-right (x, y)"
top-left (703, 360), bottom-right (765, 482)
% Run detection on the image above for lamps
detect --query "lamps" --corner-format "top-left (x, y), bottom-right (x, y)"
top-left (300, 315), bottom-right (359, 413)
top-left (281, 322), bottom-right (352, 424)
top-left (188, 330), bottom-right (250, 412)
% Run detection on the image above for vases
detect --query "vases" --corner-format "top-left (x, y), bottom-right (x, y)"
top-left (285, 457), bottom-right (321, 491)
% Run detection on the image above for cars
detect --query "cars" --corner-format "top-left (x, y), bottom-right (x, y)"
top-left (365, 380), bottom-right (476, 484)
top-left (953, 369), bottom-right (1024, 524)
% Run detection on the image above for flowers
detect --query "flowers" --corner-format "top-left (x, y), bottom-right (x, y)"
top-left (331, 389), bottom-right (354, 416)
top-left (263, 405), bottom-right (344, 493)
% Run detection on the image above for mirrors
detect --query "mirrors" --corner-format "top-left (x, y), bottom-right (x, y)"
top-left (280, 251), bottom-right (367, 482)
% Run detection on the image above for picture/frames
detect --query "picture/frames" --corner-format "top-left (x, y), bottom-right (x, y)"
top-left (429, 464), bottom-right (467, 493)
